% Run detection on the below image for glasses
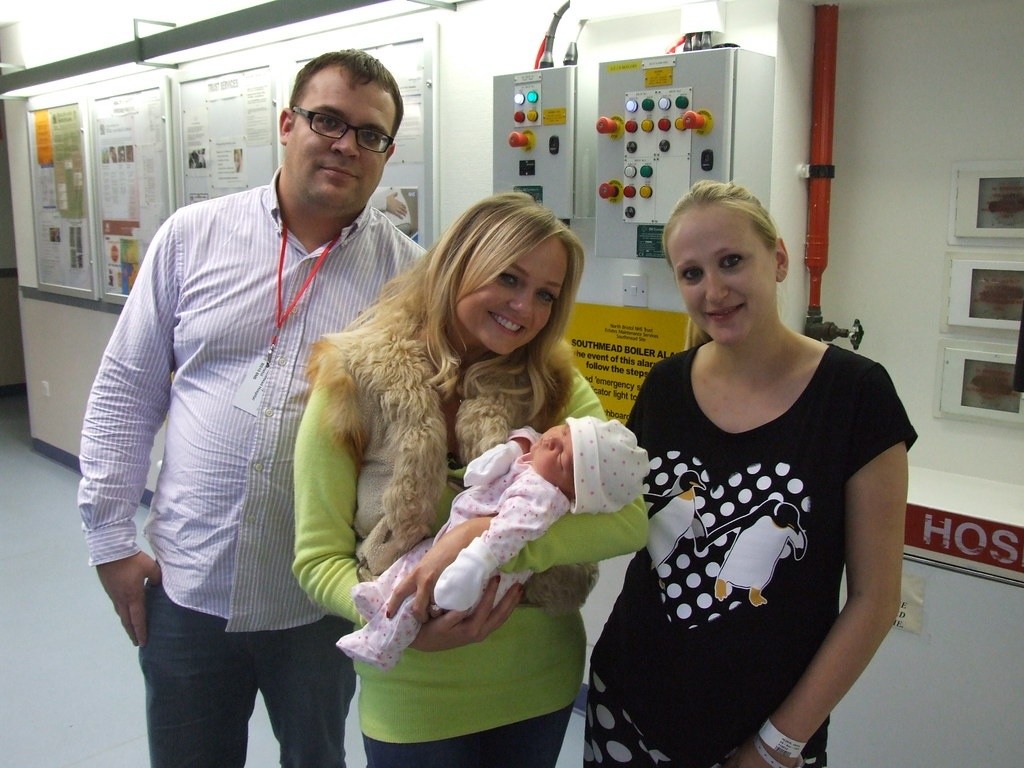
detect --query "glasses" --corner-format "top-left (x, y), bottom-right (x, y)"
top-left (292, 107), bottom-right (395, 154)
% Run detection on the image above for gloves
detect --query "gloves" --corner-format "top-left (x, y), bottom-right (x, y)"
top-left (433, 536), bottom-right (500, 612)
top-left (462, 440), bottom-right (524, 488)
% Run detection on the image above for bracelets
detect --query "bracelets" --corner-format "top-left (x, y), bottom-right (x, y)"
top-left (752, 737), bottom-right (787, 768)
top-left (759, 720), bottom-right (806, 759)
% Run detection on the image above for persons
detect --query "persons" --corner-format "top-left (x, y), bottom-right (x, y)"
top-left (79, 49), bottom-right (425, 768)
top-left (583, 182), bottom-right (921, 768)
top-left (291, 187), bottom-right (648, 768)
top-left (336, 415), bottom-right (651, 668)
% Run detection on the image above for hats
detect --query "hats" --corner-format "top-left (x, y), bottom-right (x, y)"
top-left (565, 414), bottom-right (651, 514)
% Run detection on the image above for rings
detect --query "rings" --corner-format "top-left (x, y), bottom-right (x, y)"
top-left (431, 604), bottom-right (440, 611)
